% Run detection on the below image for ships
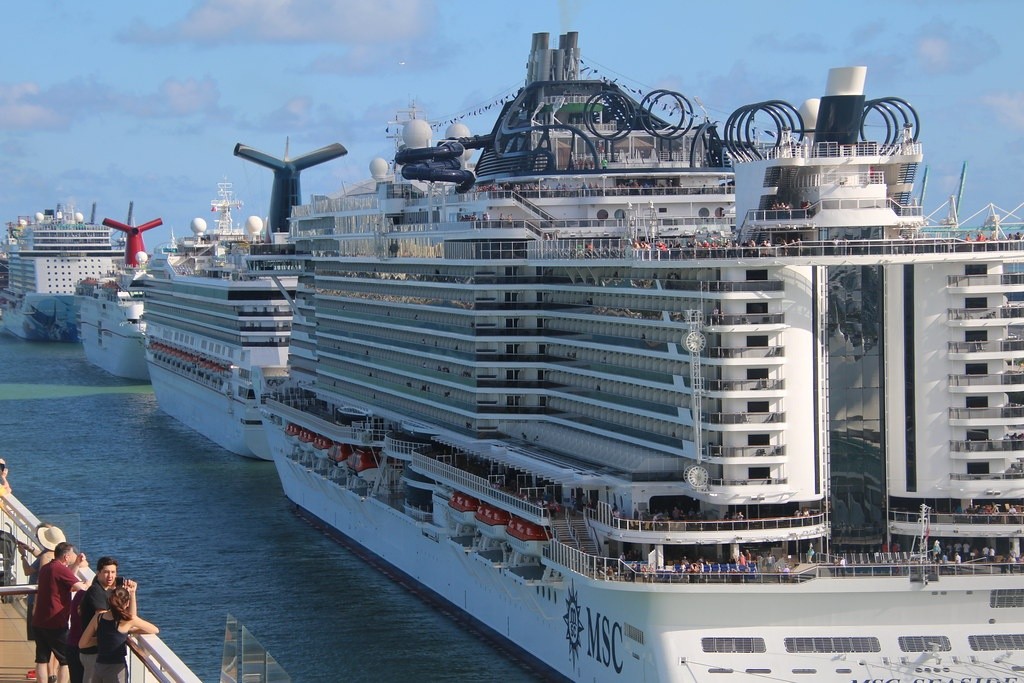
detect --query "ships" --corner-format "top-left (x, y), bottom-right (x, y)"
top-left (252, 31), bottom-right (1024, 682)
top-left (0, 205), bottom-right (126, 342)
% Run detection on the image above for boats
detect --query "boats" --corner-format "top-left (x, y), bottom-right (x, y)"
top-left (153, 228), bottom-right (179, 255)
top-left (75, 269), bottom-right (151, 382)
top-left (129, 216), bottom-right (300, 462)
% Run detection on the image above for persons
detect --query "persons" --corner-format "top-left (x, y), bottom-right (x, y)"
top-left (585, 242), bottom-right (595, 250)
top-left (595, 541), bottom-right (818, 582)
top-left (611, 502), bottom-right (621, 528)
top-left (964, 498), bottom-right (1024, 525)
top-left (759, 238), bottom-right (772, 256)
top-left (793, 507), bottom-right (812, 525)
top-left (556, 180), bottom-right (601, 196)
top-left (16, 521), bottom-right (90, 683)
top-left (66, 557), bottom-right (137, 683)
top-left (723, 237), bottom-right (740, 259)
top-left (631, 508), bottom-right (640, 521)
top-left (772, 200), bottom-right (791, 211)
top-left (742, 237), bottom-right (756, 258)
top-left (492, 468), bottom-right (561, 516)
top-left (580, 493), bottom-right (587, 506)
top-left (1001, 427), bottom-right (1024, 450)
top-left (458, 209), bottom-right (515, 227)
top-left (572, 156), bottom-right (607, 170)
top-left (0, 459), bottom-right (10, 495)
top-left (631, 237), bottom-right (718, 261)
top-left (642, 505), bottom-right (745, 530)
top-left (613, 181), bottom-right (683, 195)
top-left (476, 182), bottom-right (554, 197)
top-left (789, 238), bottom-right (804, 255)
top-left (931, 540), bottom-right (1024, 567)
top-left (966, 226), bottom-right (1024, 249)
top-left (780, 238), bottom-right (788, 256)
top-left (78, 586), bottom-right (159, 683)
top-left (881, 538), bottom-right (903, 554)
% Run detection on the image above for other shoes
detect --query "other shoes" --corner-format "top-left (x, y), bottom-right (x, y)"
top-left (48, 675), bottom-right (57, 683)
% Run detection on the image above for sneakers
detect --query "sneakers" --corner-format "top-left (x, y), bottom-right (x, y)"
top-left (26, 669), bottom-right (37, 679)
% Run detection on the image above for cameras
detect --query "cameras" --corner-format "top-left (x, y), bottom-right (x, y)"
top-left (114, 577), bottom-right (124, 587)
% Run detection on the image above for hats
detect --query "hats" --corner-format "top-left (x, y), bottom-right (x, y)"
top-left (697, 559), bottom-right (704, 562)
top-left (1011, 505), bottom-right (1014, 507)
top-left (37, 526), bottom-right (67, 551)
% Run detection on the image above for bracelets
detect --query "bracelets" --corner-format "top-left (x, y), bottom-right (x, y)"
top-left (21, 555), bottom-right (28, 559)
top-left (32, 546), bottom-right (35, 553)
